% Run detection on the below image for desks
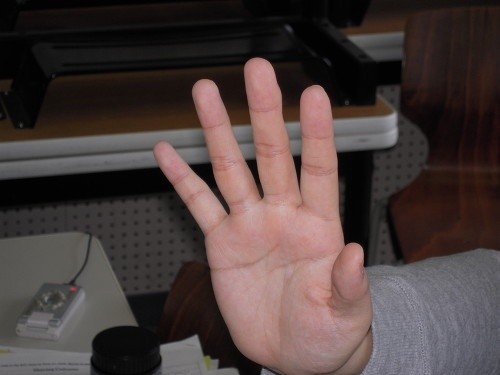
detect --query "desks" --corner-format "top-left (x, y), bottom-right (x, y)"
top-left (0, 63), bottom-right (399, 269)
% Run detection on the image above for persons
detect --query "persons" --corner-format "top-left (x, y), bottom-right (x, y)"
top-left (146, 56), bottom-right (500, 375)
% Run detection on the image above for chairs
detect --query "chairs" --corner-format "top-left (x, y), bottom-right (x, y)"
top-left (369, 1), bottom-right (500, 267)
